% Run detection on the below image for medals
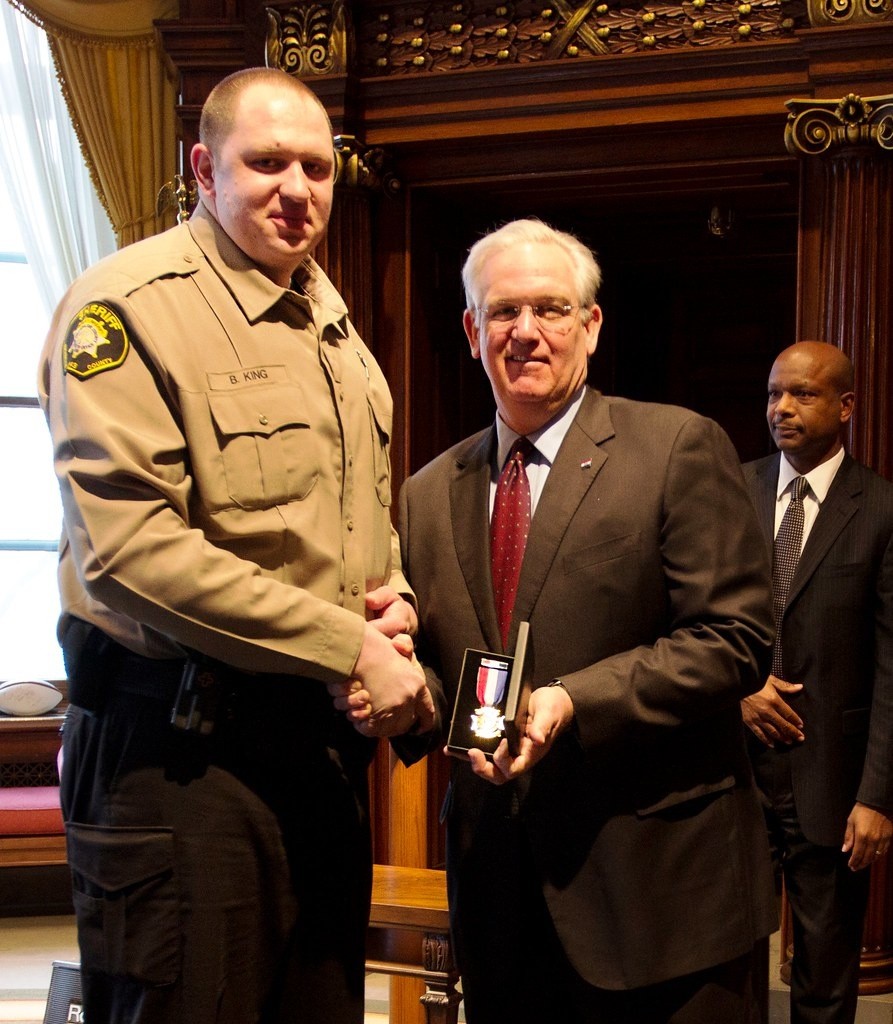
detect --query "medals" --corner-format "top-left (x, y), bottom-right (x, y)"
top-left (469, 706), bottom-right (510, 742)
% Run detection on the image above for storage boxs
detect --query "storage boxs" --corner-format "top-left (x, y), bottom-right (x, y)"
top-left (446, 620), bottom-right (534, 761)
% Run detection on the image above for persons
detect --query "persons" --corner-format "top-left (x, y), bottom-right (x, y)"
top-left (33, 65), bottom-right (432, 1024)
top-left (327, 213), bottom-right (785, 1023)
top-left (741, 338), bottom-right (893, 1023)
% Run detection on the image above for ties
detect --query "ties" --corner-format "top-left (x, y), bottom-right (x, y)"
top-left (771, 476), bottom-right (814, 680)
top-left (489, 437), bottom-right (534, 654)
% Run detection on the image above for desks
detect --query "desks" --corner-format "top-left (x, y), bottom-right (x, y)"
top-left (365, 863), bottom-right (464, 1024)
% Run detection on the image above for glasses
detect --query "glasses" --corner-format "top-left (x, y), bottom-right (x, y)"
top-left (475, 296), bottom-right (587, 324)
